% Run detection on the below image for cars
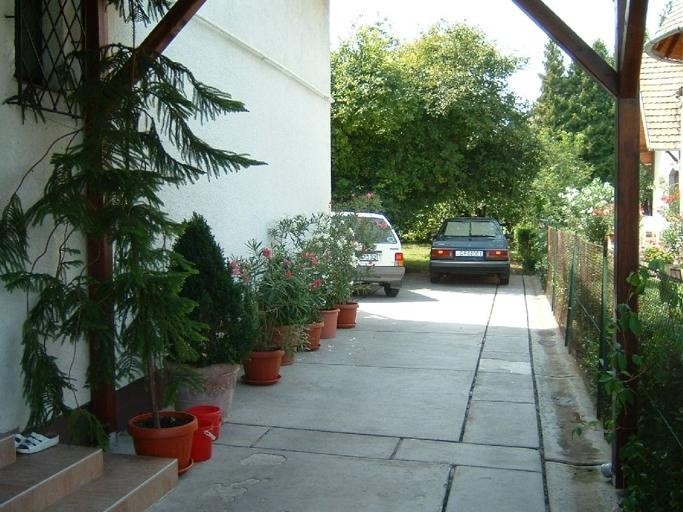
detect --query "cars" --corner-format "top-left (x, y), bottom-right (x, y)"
top-left (331, 210), bottom-right (406, 298)
top-left (429, 215), bottom-right (512, 286)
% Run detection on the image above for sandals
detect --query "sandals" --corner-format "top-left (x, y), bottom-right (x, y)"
top-left (13, 429), bottom-right (60, 455)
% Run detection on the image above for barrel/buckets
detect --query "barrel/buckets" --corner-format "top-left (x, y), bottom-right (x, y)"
top-left (180, 417), bottom-right (213, 464)
top-left (185, 403), bottom-right (224, 442)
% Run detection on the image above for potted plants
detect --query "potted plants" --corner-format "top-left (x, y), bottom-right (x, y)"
top-left (0, 41), bottom-right (265, 473)
top-left (157, 210), bottom-right (245, 423)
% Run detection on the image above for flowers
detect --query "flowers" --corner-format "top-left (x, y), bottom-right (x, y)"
top-left (230, 187), bottom-right (390, 391)
